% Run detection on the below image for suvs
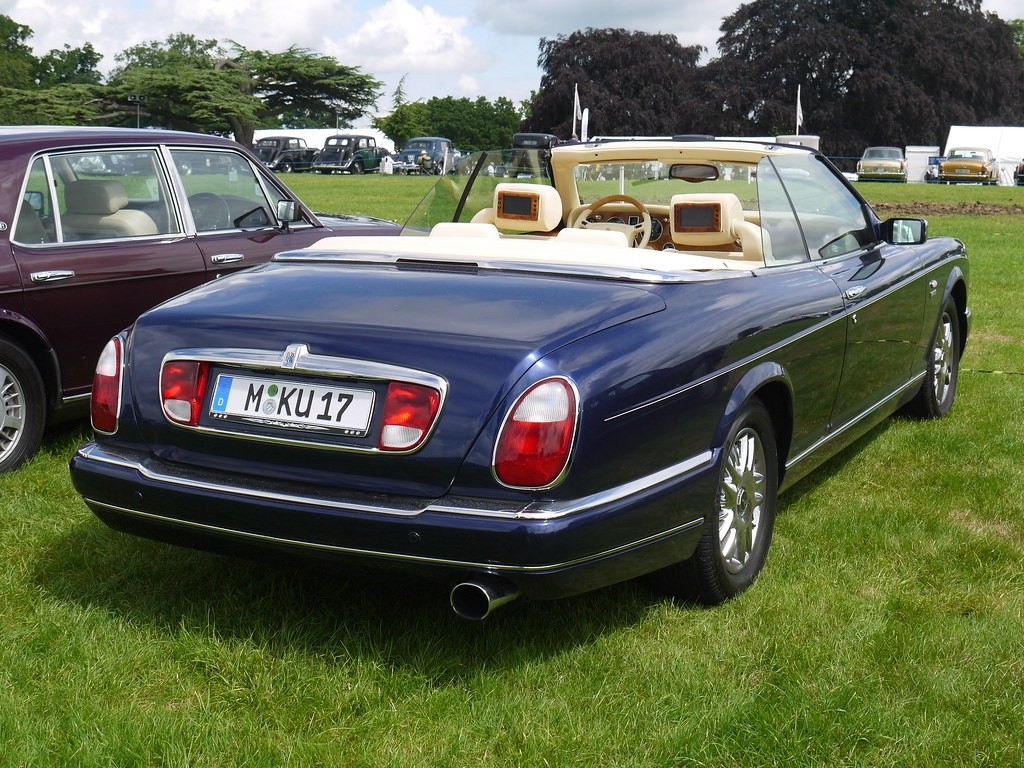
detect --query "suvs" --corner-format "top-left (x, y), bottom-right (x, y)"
top-left (507, 132), bottom-right (562, 178)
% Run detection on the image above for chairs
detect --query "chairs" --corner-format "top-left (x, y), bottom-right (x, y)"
top-left (58, 178), bottom-right (158, 238)
top-left (669, 192), bottom-right (775, 261)
top-left (419, 158), bottom-right (437, 176)
top-left (471, 182), bottom-right (562, 238)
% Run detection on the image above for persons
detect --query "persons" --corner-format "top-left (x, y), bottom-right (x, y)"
top-left (417, 150), bottom-right (431, 173)
top-left (926, 166), bottom-right (939, 184)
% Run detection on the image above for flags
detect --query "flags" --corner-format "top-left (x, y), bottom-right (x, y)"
top-left (797, 100), bottom-right (804, 126)
top-left (576, 91), bottom-right (581, 120)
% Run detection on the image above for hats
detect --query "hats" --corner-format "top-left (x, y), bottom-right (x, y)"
top-left (420, 150), bottom-right (428, 156)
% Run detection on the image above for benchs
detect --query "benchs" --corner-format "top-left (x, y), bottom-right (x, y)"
top-left (311, 223), bottom-right (749, 269)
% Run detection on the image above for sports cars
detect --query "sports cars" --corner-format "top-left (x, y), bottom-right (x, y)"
top-left (67, 136), bottom-right (973, 621)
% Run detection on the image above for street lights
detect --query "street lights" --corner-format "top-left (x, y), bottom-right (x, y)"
top-left (335, 107), bottom-right (343, 129)
top-left (127, 94), bottom-right (146, 128)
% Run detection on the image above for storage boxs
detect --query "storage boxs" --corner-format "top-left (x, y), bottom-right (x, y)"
top-left (379, 161), bottom-right (393, 173)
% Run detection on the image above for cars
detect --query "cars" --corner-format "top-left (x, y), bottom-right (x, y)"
top-left (396, 137), bottom-right (473, 177)
top-left (100, 150), bottom-right (239, 178)
top-left (1, 122), bottom-right (431, 478)
top-left (238, 136), bottom-right (322, 174)
top-left (309, 133), bottom-right (400, 175)
top-left (938, 144), bottom-right (999, 185)
top-left (855, 146), bottom-right (909, 184)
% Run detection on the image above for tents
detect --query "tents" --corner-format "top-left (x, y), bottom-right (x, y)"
top-left (943, 125), bottom-right (1024, 186)
